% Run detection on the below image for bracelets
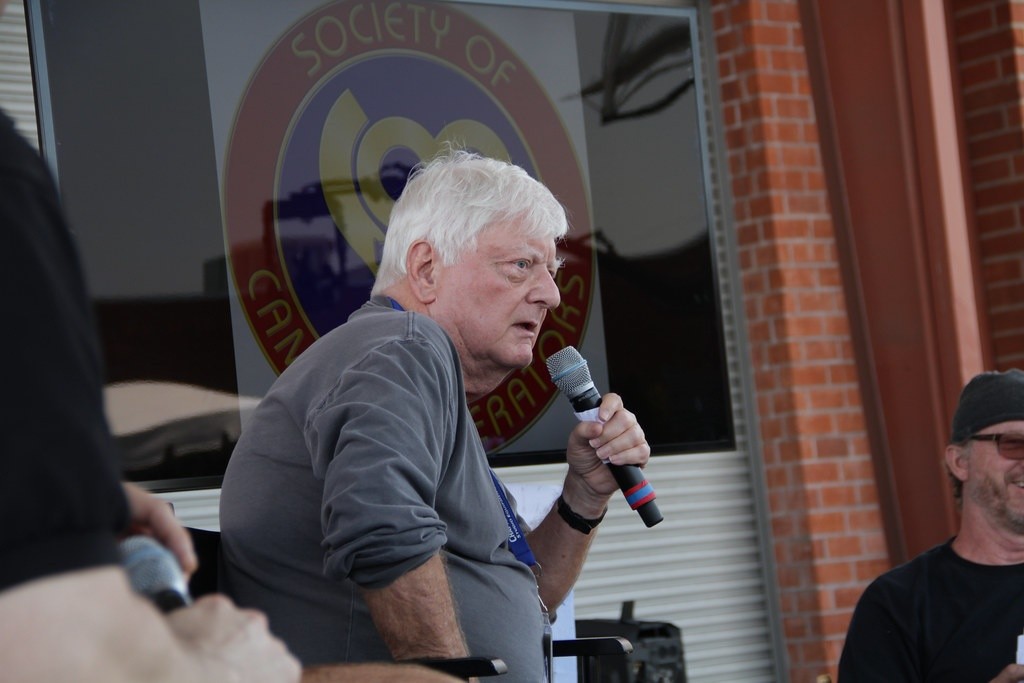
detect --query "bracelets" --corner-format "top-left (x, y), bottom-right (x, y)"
top-left (557, 494), bottom-right (609, 535)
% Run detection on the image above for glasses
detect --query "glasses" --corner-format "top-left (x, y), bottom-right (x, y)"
top-left (963, 432), bottom-right (1024, 459)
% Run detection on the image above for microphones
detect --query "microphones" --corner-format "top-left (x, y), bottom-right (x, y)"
top-left (116, 534), bottom-right (190, 616)
top-left (546, 345), bottom-right (664, 528)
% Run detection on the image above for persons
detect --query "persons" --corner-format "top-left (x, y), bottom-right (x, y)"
top-left (836, 367), bottom-right (1024, 682)
top-left (0, 106), bottom-right (305, 682)
top-left (220, 116), bottom-right (650, 683)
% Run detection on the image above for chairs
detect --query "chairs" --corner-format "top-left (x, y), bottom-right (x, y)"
top-left (168, 481), bottom-right (638, 683)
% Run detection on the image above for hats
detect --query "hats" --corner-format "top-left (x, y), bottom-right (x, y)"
top-left (951, 369), bottom-right (1024, 439)
